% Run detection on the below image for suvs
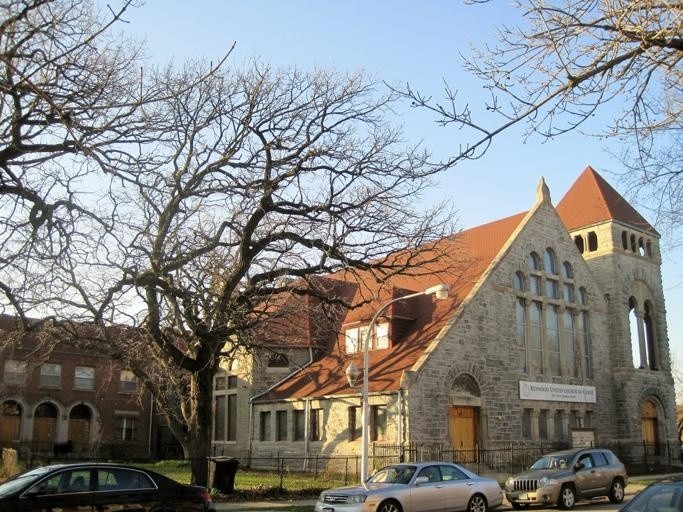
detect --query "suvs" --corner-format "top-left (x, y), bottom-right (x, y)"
top-left (505, 447), bottom-right (628, 509)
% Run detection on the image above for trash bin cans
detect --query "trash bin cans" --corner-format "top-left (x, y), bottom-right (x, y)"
top-left (206, 456), bottom-right (241, 496)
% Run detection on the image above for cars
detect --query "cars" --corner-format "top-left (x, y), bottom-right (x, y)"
top-left (0, 462), bottom-right (214, 509)
top-left (313, 460), bottom-right (504, 511)
top-left (617, 472), bottom-right (683, 512)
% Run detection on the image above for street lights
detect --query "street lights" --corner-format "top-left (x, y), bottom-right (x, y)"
top-left (343, 283), bottom-right (450, 486)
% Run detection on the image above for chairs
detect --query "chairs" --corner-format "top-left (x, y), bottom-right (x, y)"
top-left (57, 474), bottom-right (71, 493)
top-left (69, 476), bottom-right (85, 492)
top-left (118, 476), bottom-right (137, 489)
